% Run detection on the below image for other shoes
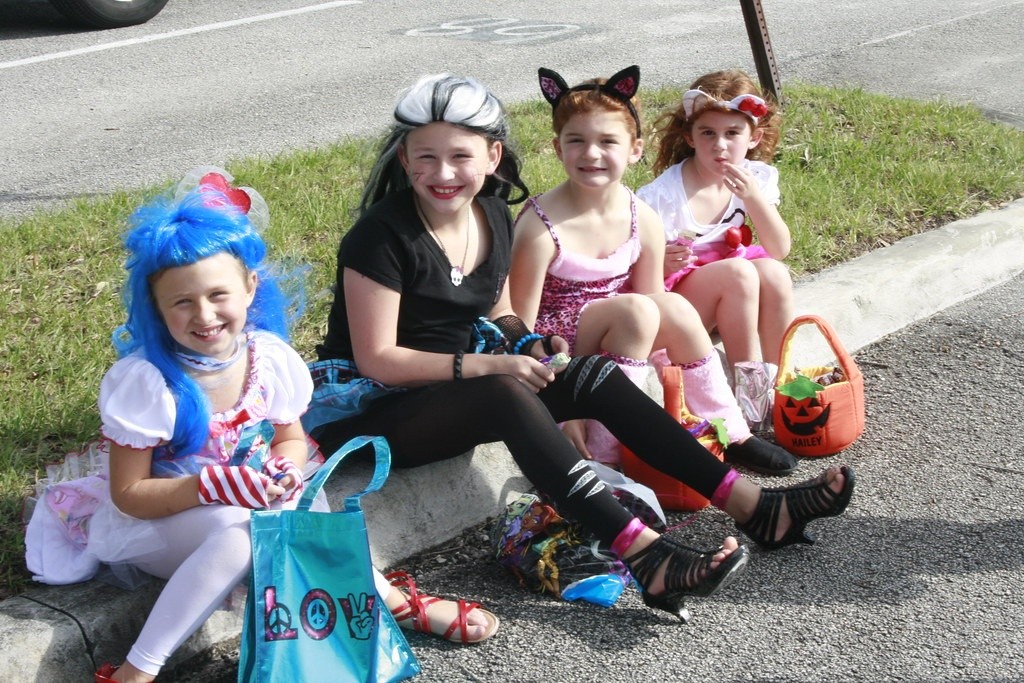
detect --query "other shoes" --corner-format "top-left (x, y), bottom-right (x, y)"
top-left (723, 434), bottom-right (799, 476)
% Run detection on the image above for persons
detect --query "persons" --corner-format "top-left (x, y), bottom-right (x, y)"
top-left (96, 204), bottom-right (500, 683)
top-left (301, 74), bottom-right (856, 624)
top-left (637, 69), bottom-right (795, 435)
top-left (510, 77), bottom-right (800, 476)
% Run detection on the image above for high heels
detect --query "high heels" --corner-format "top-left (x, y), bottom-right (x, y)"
top-left (622, 534), bottom-right (750, 624)
top-left (734, 464), bottom-right (856, 551)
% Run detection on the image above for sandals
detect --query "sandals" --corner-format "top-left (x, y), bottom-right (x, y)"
top-left (95, 663), bottom-right (155, 683)
top-left (384, 571), bottom-right (500, 645)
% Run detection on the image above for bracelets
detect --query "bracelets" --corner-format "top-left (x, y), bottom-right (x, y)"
top-left (453, 350), bottom-right (463, 383)
top-left (513, 333), bottom-right (544, 355)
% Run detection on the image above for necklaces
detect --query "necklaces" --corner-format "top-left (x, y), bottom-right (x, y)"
top-left (420, 205), bottom-right (471, 287)
